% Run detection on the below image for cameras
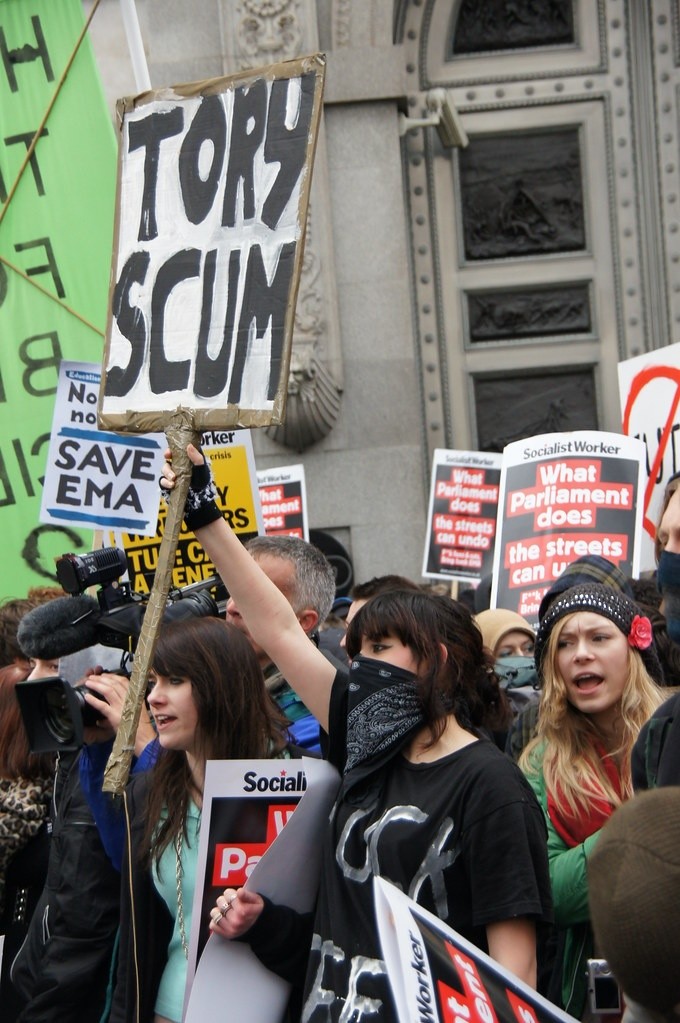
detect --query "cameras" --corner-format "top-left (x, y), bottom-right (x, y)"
top-left (583, 958), bottom-right (624, 1014)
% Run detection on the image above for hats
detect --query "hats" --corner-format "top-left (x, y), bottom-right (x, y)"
top-left (534, 583), bottom-right (664, 689)
top-left (588, 786), bottom-right (680, 1011)
top-left (470, 609), bottom-right (538, 663)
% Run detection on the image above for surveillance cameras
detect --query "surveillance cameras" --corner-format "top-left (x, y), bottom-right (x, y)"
top-left (425, 86), bottom-right (469, 149)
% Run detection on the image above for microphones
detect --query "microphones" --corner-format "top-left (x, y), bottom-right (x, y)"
top-left (16, 594), bottom-right (106, 660)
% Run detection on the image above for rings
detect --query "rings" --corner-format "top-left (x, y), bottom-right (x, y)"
top-left (213, 913), bottom-right (224, 925)
top-left (228, 895), bottom-right (238, 905)
top-left (220, 902), bottom-right (232, 917)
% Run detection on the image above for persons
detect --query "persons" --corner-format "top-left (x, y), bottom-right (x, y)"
top-left (109, 617), bottom-right (317, 1023)
top-left (159, 442), bottom-right (553, 1023)
top-left (0, 537), bottom-right (680, 1023)
top-left (519, 584), bottom-right (664, 1023)
top-left (587, 788), bottom-right (680, 1023)
top-left (631, 476), bottom-right (680, 795)
